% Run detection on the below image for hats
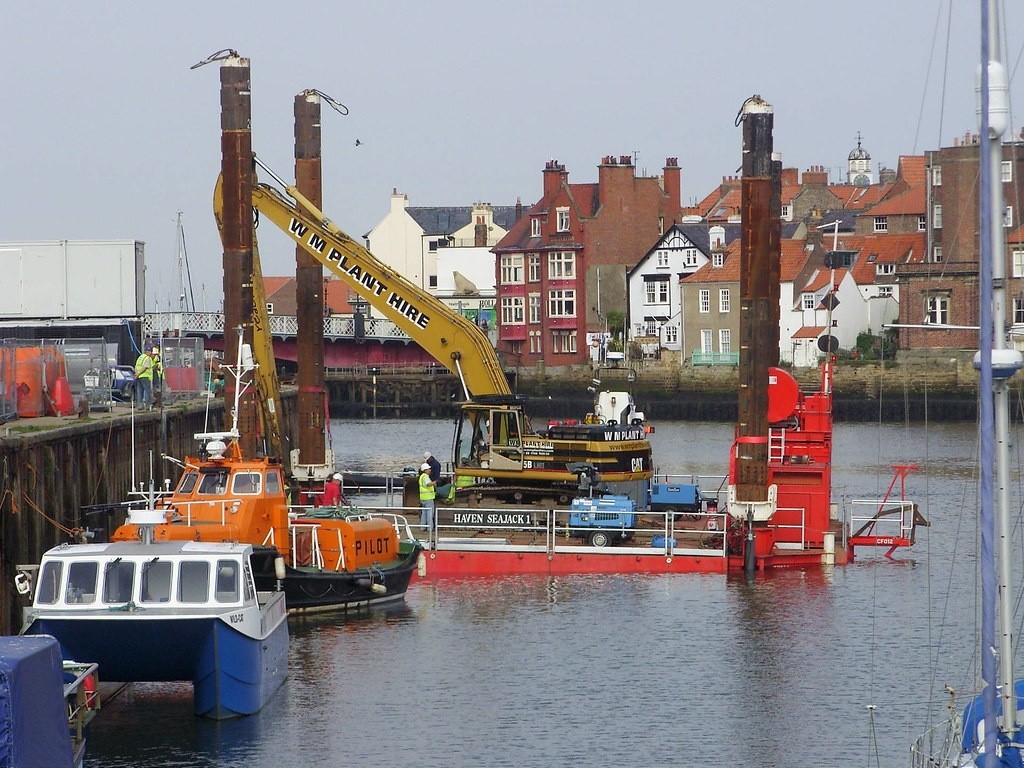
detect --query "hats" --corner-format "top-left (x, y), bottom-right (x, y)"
top-left (332, 473), bottom-right (342, 481)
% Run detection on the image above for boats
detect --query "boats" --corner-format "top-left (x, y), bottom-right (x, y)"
top-left (142, 45), bottom-right (941, 576)
top-left (13, 537), bottom-right (291, 721)
top-left (99, 325), bottom-right (425, 621)
top-left (886, 0), bottom-right (1023, 768)
top-left (0, 629), bottom-right (103, 768)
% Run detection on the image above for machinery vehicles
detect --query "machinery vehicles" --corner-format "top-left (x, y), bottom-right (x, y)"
top-left (211, 149), bottom-right (709, 550)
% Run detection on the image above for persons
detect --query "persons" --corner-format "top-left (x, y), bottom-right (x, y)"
top-left (133, 345), bottom-right (166, 412)
top-left (420, 463), bottom-right (438, 531)
top-left (284, 484), bottom-right (292, 511)
top-left (322, 473), bottom-right (343, 506)
top-left (447, 457), bottom-right (475, 503)
top-left (423, 451), bottom-right (441, 492)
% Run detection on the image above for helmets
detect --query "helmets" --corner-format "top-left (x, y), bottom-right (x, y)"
top-left (420, 463), bottom-right (431, 470)
top-left (423, 452), bottom-right (431, 462)
top-left (144, 346), bottom-right (151, 352)
top-left (151, 348), bottom-right (159, 354)
